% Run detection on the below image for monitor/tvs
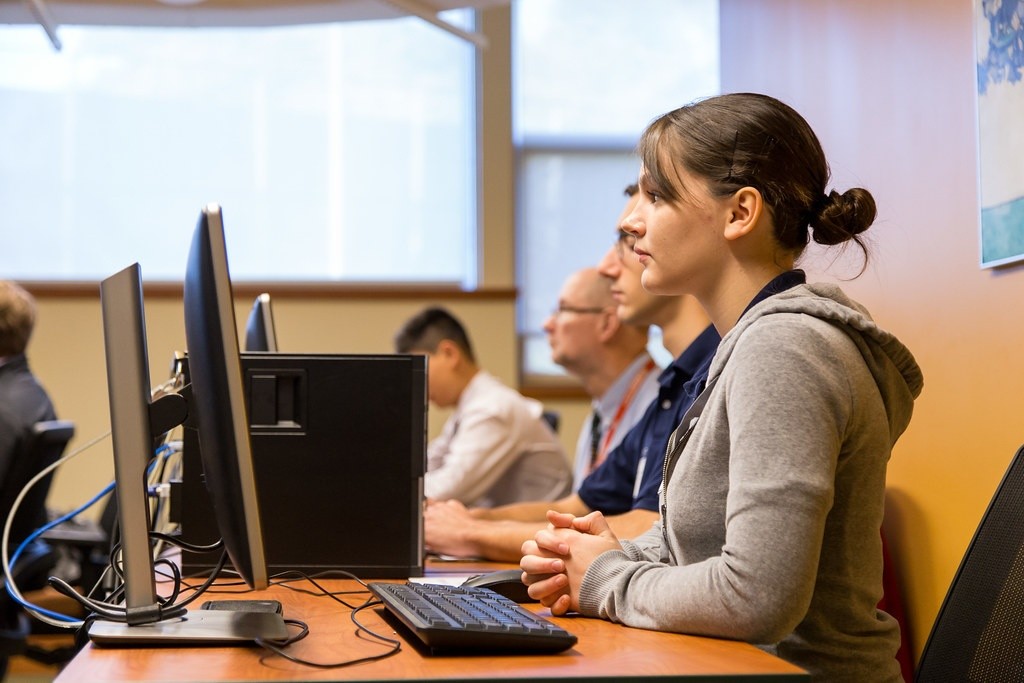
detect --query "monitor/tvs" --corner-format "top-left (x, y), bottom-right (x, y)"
top-left (183, 204), bottom-right (271, 591)
top-left (245, 292), bottom-right (278, 351)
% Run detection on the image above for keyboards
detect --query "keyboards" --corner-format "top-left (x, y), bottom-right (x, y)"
top-left (367, 582), bottom-right (578, 657)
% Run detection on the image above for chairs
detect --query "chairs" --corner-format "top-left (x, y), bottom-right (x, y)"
top-left (1, 419), bottom-right (75, 682)
top-left (909, 444), bottom-right (1024, 683)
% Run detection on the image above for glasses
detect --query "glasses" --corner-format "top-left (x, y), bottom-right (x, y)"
top-left (550, 304), bottom-right (604, 321)
top-left (615, 233), bottom-right (635, 258)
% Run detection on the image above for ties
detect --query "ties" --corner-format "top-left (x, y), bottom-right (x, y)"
top-left (590, 412), bottom-right (602, 473)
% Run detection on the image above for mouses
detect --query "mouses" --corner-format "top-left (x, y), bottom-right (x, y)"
top-left (459, 570), bottom-right (541, 603)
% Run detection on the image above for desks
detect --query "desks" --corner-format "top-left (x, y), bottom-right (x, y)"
top-left (49, 556), bottom-right (812, 683)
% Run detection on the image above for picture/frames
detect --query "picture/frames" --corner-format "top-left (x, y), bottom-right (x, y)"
top-left (972, 0), bottom-right (1024, 271)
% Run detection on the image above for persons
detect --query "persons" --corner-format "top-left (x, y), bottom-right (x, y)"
top-left (520, 91), bottom-right (928, 682)
top-left (542, 263), bottom-right (665, 525)
top-left (421, 177), bottom-right (724, 562)
top-left (390, 305), bottom-right (573, 509)
top-left (0, 278), bottom-right (60, 562)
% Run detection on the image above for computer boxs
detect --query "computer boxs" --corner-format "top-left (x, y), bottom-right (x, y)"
top-left (183, 350), bottom-right (430, 579)
